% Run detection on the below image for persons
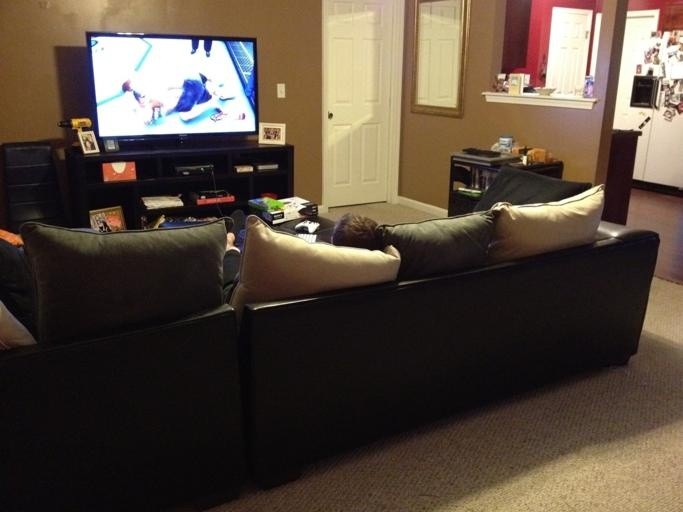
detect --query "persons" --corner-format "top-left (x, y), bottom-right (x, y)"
top-left (191, 38), bottom-right (212, 57)
top-left (264, 129), bottom-right (281, 139)
top-left (223, 212), bottom-right (379, 304)
top-left (96, 220), bottom-right (112, 233)
top-left (165, 72), bottom-right (212, 116)
top-left (84, 134), bottom-right (95, 150)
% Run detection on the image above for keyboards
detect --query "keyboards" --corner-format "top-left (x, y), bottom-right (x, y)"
top-left (296, 233), bottom-right (317, 243)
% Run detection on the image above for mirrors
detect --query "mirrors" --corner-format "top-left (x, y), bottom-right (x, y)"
top-left (409, 0), bottom-right (470, 122)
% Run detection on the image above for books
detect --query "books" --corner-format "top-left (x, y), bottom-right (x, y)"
top-left (234, 165), bottom-right (254, 173)
top-left (454, 162), bottom-right (498, 197)
top-left (257, 163), bottom-right (279, 170)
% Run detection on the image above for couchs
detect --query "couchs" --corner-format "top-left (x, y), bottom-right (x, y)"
top-left (0, 219), bottom-right (661, 509)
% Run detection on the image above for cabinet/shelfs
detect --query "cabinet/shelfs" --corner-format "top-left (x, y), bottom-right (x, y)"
top-left (5, 137), bottom-right (295, 222)
top-left (446, 148), bottom-right (562, 217)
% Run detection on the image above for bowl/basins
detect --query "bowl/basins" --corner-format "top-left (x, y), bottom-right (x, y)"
top-left (533, 87), bottom-right (556, 96)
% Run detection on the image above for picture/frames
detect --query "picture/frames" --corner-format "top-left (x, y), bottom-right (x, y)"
top-left (77, 130), bottom-right (100, 154)
top-left (256, 122), bottom-right (286, 146)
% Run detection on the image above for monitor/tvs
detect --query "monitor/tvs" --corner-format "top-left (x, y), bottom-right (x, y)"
top-left (85, 31), bottom-right (259, 151)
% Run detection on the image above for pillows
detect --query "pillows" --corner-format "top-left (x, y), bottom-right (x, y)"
top-left (485, 183), bottom-right (605, 267)
top-left (331, 208), bottom-right (502, 282)
top-left (18, 218), bottom-right (228, 342)
top-left (228, 215), bottom-right (402, 331)
top-left (470, 164), bottom-right (592, 212)
top-left (0, 234), bottom-right (36, 338)
